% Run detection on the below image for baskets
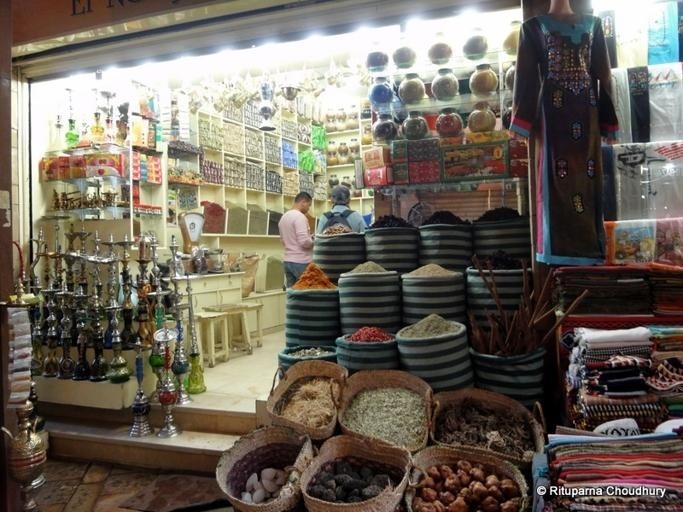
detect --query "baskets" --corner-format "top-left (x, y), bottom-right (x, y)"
top-left (216, 360), bottom-right (546, 512)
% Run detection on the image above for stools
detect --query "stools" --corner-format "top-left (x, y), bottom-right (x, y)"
top-left (193, 311), bottom-right (228, 369)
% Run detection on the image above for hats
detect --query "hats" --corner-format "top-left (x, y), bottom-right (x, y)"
top-left (329, 185), bottom-right (350, 199)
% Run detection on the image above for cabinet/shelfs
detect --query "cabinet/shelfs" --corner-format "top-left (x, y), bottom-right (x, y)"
top-left (28, 142), bottom-right (164, 410)
top-left (164, 100), bottom-right (375, 354)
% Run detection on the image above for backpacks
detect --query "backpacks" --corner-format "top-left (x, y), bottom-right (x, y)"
top-left (322, 209), bottom-right (356, 230)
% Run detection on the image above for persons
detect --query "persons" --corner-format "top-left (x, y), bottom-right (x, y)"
top-left (509, 0), bottom-right (619, 265)
top-left (277, 191), bottom-right (314, 289)
top-left (317, 185), bottom-right (365, 233)
